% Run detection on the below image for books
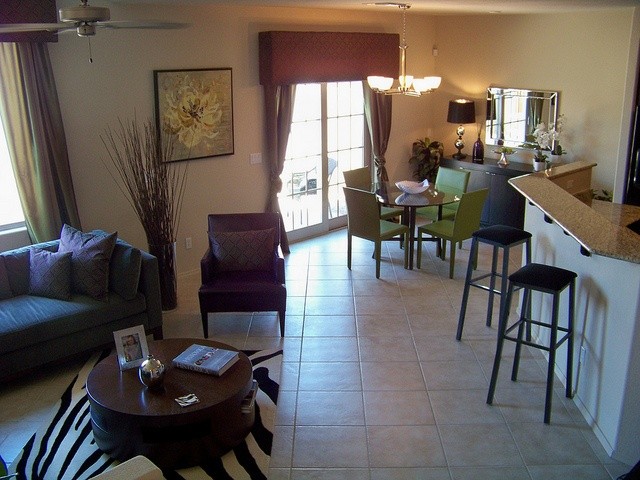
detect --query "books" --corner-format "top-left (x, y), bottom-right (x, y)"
top-left (172, 344), bottom-right (240, 376)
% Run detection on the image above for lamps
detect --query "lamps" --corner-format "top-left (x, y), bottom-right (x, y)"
top-left (447, 99), bottom-right (475, 159)
top-left (367, 9), bottom-right (441, 98)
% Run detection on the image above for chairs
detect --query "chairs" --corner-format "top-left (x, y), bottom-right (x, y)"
top-left (343, 166), bottom-right (405, 258)
top-left (409, 166), bottom-right (471, 249)
top-left (417, 189), bottom-right (489, 279)
top-left (198, 212), bottom-right (286, 339)
top-left (342, 186), bottom-right (409, 279)
top-left (82, 455), bottom-right (166, 480)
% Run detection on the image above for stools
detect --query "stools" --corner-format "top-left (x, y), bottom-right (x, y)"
top-left (487, 263), bottom-right (578, 423)
top-left (455, 224), bottom-right (532, 342)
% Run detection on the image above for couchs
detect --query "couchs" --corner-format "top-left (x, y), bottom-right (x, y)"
top-left (0, 228), bottom-right (163, 379)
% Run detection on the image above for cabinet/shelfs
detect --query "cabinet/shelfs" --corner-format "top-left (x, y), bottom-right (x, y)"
top-left (439, 149), bottom-right (557, 231)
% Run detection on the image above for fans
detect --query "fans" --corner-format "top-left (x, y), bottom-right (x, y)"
top-left (0, 0), bottom-right (193, 63)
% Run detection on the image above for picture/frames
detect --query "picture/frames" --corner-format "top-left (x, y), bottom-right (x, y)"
top-left (153, 67), bottom-right (234, 163)
top-left (113, 324), bottom-right (150, 370)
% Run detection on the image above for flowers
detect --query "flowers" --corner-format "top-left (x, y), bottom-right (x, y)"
top-left (548, 114), bottom-right (568, 154)
top-left (519, 121), bottom-right (554, 162)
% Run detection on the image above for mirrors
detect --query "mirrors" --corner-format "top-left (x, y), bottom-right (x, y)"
top-left (484, 80), bottom-right (558, 151)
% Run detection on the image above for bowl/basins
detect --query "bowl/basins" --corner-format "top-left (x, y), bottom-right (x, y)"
top-left (395, 178), bottom-right (430, 195)
top-left (395, 193), bottom-right (430, 206)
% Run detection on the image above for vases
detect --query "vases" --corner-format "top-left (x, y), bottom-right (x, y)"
top-left (472, 137), bottom-right (484, 163)
top-left (146, 242), bottom-right (176, 311)
top-left (550, 154), bottom-right (562, 165)
top-left (532, 159), bottom-right (546, 171)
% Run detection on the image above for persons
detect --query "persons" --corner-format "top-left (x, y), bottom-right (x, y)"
top-left (124, 335), bottom-right (144, 362)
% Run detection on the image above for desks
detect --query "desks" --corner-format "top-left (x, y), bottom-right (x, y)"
top-left (374, 180), bottom-right (461, 270)
top-left (86, 338), bottom-right (258, 470)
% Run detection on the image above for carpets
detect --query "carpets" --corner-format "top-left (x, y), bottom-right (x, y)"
top-left (6, 336), bottom-right (282, 479)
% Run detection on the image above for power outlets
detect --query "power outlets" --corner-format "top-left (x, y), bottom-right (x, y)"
top-left (185, 237), bottom-right (192, 248)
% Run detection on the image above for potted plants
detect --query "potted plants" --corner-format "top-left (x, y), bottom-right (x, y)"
top-left (497, 131), bottom-right (504, 145)
top-left (491, 147), bottom-right (516, 165)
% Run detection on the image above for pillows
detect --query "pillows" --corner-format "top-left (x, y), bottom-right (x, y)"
top-left (109, 245), bottom-right (142, 300)
top-left (57, 224), bottom-right (118, 303)
top-left (28, 246), bottom-right (73, 302)
